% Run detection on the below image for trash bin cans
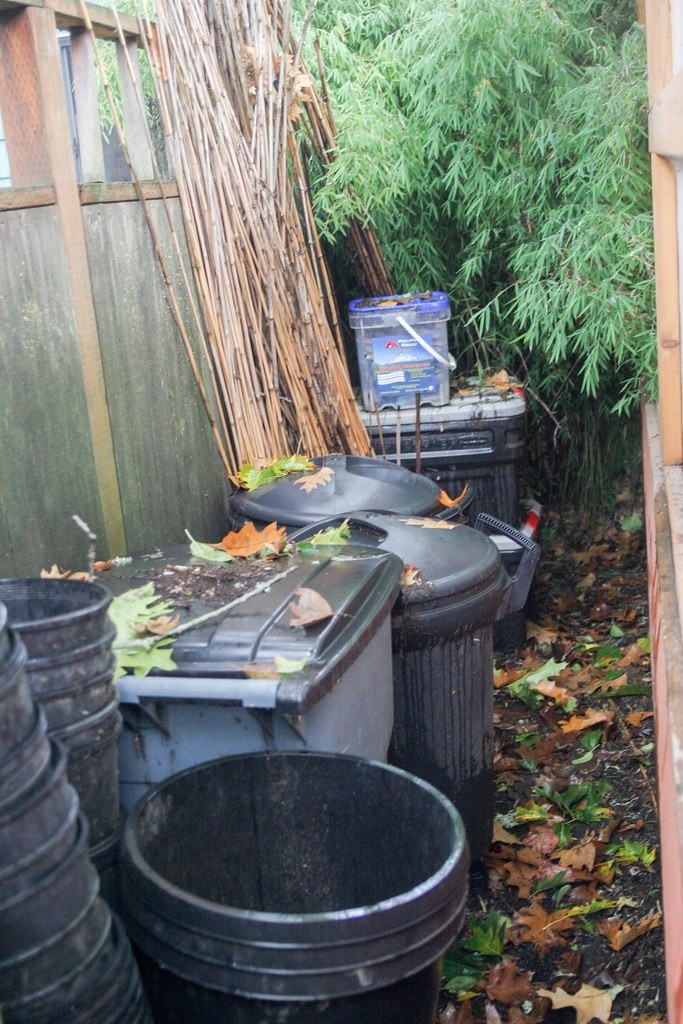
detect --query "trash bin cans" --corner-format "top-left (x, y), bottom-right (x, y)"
top-left (117, 748), bottom-right (470, 1024)
top-left (0, 601), bottom-right (152, 1024)
top-left (230, 454), bottom-right (441, 542)
top-left (0, 576), bottom-right (122, 909)
top-left (289, 507), bottom-right (543, 910)
top-left (100, 542), bottom-right (405, 811)
top-left (352, 373), bottom-right (528, 537)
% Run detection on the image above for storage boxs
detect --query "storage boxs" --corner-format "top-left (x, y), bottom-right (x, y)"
top-left (347, 289), bottom-right (457, 413)
